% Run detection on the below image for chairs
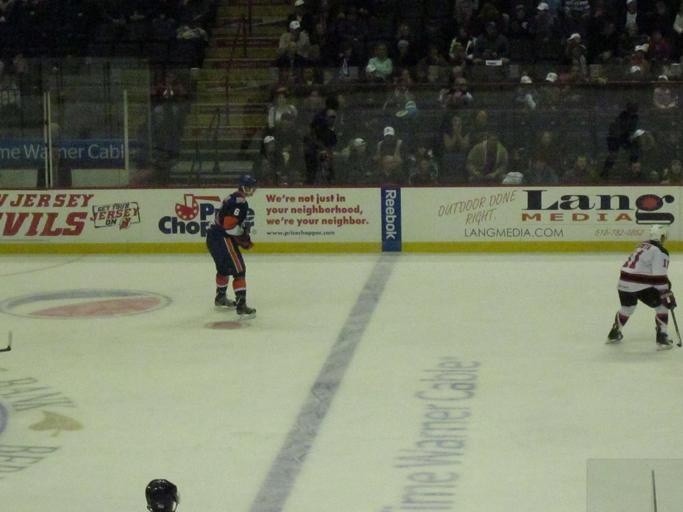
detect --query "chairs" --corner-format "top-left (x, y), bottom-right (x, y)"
top-left (0, 54), bottom-right (150, 140)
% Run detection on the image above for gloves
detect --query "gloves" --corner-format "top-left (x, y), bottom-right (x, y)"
top-left (662, 290), bottom-right (678, 310)
top-left (233, 230), bottom-right (251, 249)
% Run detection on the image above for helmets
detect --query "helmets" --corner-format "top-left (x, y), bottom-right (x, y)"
top-left (239, 175), bottom-right (256, 196)
top-left (650, 224), bottom-right (668, 245)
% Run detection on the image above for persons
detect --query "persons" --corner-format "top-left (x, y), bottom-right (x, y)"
top-left (252, 0), bottom-right (683, 190)
top-left (1, 1), bottom-right (214, 188)
top-left (204, 171), bottom-right (257, 316)
top-left (605, 224), bottom-right (678, 346)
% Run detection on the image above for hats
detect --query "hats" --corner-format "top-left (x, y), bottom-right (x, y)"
top-left (520, 76), bottom-right (532, 84)
top-left (384, 126), bottom-right (394, 136)
top-left (538, 3), bottom-right (549, 10)
top-left (405, 101), bottom-right (416, 108)
top-left (289, 21), bottom-right (301, 28)
top-left (630, 66), bottom-right (640, 73)
top-left (635, 46), bottom-right (648, 53)
top-left (295, 0), bottom-right (304, 5)
top-left (659, 74), bottom-right (668, 81)
top-left (264, 136), bottom-right (274, 143)
top-left (354, 138), bottom-right (367, 146)
top-left (569, 33), bottom-right (580, 40)
top-left (631, 130), bottom-right (646, 141)
top-left (546, 72), bottom-right (558, 82)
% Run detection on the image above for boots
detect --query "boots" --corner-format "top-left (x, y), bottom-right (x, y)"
top-left (607, 324), bottom-right (623, 341)
top-left (215, 288), bottom-right (236, 305)
top-left (656, 326), bottom-right (673, 346)
top-left (236, 292), bottom-right (256, 314)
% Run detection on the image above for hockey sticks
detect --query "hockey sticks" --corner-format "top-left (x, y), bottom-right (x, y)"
top-left (671, 307), bottom-right (682, 347)
top-left (0, 329), bottom-right (12, 352)
